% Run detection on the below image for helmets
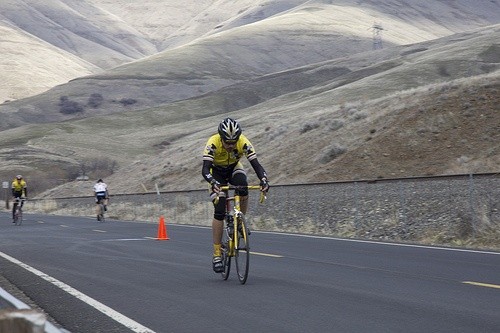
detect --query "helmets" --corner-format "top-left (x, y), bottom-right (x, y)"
top-left (16, 174), bottom-right (22, 179)
top-left (218, 118), bottom-right (241, 140)
top-left (96, 178), bottom-right (103, 183)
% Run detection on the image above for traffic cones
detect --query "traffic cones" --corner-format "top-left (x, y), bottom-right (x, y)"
top-left (158, 215), bottom-right (171, 240)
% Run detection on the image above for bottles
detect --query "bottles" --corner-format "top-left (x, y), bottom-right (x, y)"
top-left (225, 215), bottom-right (234, 239)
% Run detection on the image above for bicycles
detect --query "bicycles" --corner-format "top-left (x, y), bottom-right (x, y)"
top-left (95, 198), bottom-right (109, 221)
top-left (13, 199), bottom-right (28, 226)
top-left (216, 186), bottom-right (267, 283)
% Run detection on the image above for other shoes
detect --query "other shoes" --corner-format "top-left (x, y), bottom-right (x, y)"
top-left (104, 207), bottom-right (107, 211)
top-left (97, 215), bottom-right (100, 220)
top-left (13, 218), bottom-right (15, 223)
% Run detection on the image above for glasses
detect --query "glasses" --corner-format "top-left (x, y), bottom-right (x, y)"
top-left (222, 138), bottom-right (238, 145)
top-left (17, 178), bottom-right (21, 180)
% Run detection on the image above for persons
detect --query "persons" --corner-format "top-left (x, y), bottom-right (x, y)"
top-left (93, 178), bottom-right (109, 221)
top-left (11, 175), bottom-right (28, 222)
top-left (201, 117), bottom-right (269, 272)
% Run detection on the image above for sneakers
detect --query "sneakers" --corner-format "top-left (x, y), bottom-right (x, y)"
top-left (213, 256), bottom-right (224, 273)
top-left (238, 223), bottom-right (251, 236)
